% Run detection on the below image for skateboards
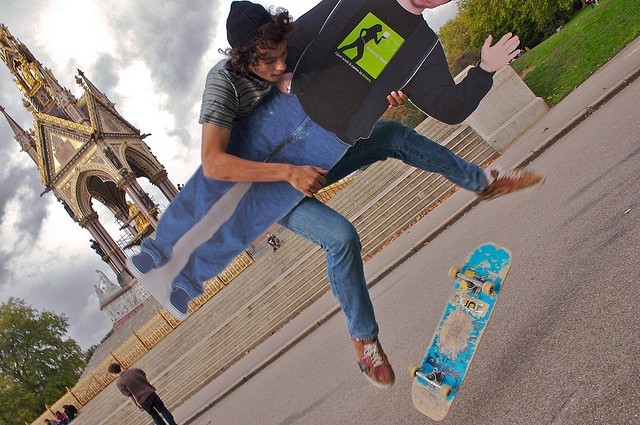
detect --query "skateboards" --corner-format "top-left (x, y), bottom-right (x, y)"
top-left (408, 241), bottom-right (513, 420)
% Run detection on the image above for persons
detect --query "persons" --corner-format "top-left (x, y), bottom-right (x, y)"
top-left (265, 232), bottom-right (280, 251)
top-left (585, 0), bottom-right (594, 9)
top-left (200, 1), bottom-right (548, 391)
top-left (108, 362), bottom-right (178, 424)
top-left (56, 411), bottom-right (70, 425)
top-left (127, 200), bottom-right (149, 231)
top-left (44, 419), bottom-right (58, 425)
top-left (90, 238), bottom-right (108, 262)
top-left (177, 183), bottom-right (185, 191)
top-left (63, 405), bottom-right (77, 419)
top-left (593, 0), bottom-right (599, 5)
top-left (131, 2), bottom-right (522, 320)
top-left (338, 24), bottom-right (384, 60)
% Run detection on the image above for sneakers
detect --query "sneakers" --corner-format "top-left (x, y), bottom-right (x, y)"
top-left (128, 248), bottom-right (159, 274)
top-left (165, 277), bottom-right (191, 322)
top-left (479, 169), bottom-right (545, 202)
top-left (350, 334), bottom-right (396, 389)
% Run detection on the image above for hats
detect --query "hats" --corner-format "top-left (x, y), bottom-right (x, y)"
top-left (226, 2), bottom-right (279, 48)
